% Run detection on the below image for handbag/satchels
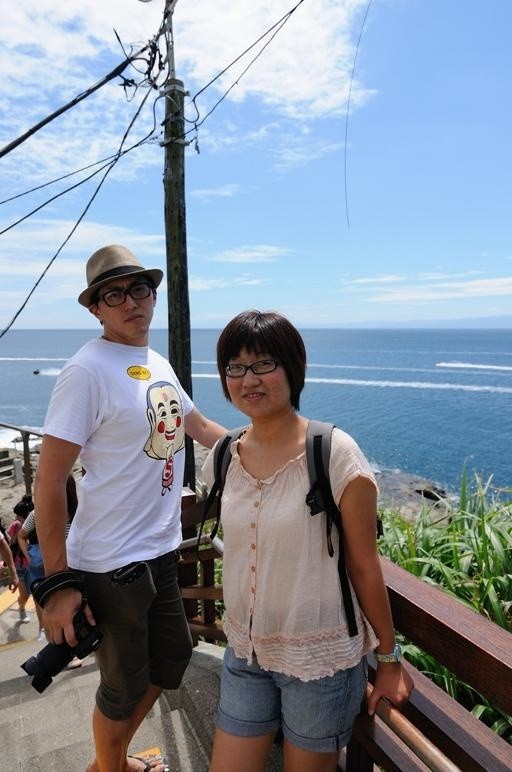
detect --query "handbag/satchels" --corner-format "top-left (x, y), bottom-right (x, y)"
top-left (3, 544), bottom-right (18, 567)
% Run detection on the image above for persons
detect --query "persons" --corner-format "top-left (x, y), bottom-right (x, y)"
top-left (30, 243), bottom-right (228, 771)
top-left (199, 311), bottom-right (414, 772)
top-left (0, 472), bottom-right (84, 670)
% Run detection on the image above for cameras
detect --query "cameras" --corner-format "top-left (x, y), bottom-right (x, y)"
top-left (20, 613), bottom-right (104, 696)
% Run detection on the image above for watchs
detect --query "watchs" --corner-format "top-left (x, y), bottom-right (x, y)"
top-left (373, 643), bottom-right (402, 663)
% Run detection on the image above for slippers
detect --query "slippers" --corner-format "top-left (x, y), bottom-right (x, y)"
top-left (85, 754), bottom-right (170, 772)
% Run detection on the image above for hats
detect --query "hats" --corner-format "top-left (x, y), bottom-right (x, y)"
top-left (78, 245), bottom-right (163, 308)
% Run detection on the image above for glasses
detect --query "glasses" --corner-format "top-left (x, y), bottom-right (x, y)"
top-left (95, 282), bottom-right (154, 307)
top-left (222, 359), bottom-right (281, 378)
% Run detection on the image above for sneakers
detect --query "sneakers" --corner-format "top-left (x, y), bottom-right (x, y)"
top-left (39, 625), bottom-right (46, 640)
top-left (19, 607), bottom-right (30, 623)
